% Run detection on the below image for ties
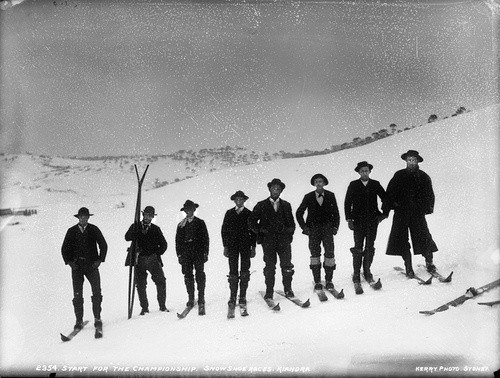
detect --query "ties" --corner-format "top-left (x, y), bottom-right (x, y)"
top-left (185, 219), bottom-right (189, 225)
top-left (82, 227), bottom-right (84, 233)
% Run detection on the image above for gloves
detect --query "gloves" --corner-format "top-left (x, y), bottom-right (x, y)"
top-left (347, 219), bottom-right (354, 230)
top-left (69, 260), bottom-right (78, 271)
top-left (147, 253), bottom-right (159, 262)
top-left (249, 249), bottom-right (255, 258)
top-left (376, 213), bottom-right (387, 222)
top-left (201, 255), bottom-right (208, 264)
top-left (286, 228), bottom-right (294, 235)
top-left (259, 225), bottom-right (270, 233)
top-left (135, 233), bottom-right (144, 242)
top-left (333, 227), bottom-right (337, 235)
top-left (89, 259), bottom-right (102, 271)
top-left (177, 256), bottom-right (182, 265)
top-left (223, 246), bottom-right (230, 258)
top-left (304, 225), bottom-right (312, 234)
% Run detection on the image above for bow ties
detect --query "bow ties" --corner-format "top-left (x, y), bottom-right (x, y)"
top-left (318, 194), bottom-right (323, 198)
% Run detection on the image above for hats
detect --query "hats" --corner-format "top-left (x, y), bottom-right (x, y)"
top-left (180, 200), bottom-right (199, 211)
top-left (311, 174), bottom-right (328, 186)
top-left (231, 191), bottom-right (249, 200)
top-left (140, 206), bottom-right (158, 215)
top-left (401, 150), bottom-right (423, 162)
top-left (354, 161), bottom-right (373, 171)
top-left (74, 207), bottom-right (93, 217)
top-left (267, 179), bottom-right (285, 189)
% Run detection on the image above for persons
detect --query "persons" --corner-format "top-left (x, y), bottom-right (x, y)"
top-left (295, 172), bottom-right (340, 291)
top-left (343, 160), bottom-right (386, 284)
top-left (250, 178), bottom-right (296, 300)
top-left (221, 190), bottom-right (257, 309)
top-left (380, 149), bottom-right (439, 279)
top-left (61, 207), bottom-right (108, 330)
top-left (174, 199), bottom-right (210, 308)
top-left (123, 205), bottom-right (170, 316)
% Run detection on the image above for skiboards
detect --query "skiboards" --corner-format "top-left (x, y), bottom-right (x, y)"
top-left (60, 320), bottom-right (103, 342)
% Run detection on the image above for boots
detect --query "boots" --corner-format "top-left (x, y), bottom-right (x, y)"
top-left (157, 281), bottom-right (169, 312)
top-left (402, 253), bottom-right (414, 277)
top-left (282, 266), bottom-right (294, 296)
top-left (184, 276), bottom-right (195, 307)
top-left (196, 273), bottom-right (206, 303)
top-left (324, 264), bottom-right (336, 289)
top-left (423, 252), bottom-right (433, 272)
top-left (240, 271), bottom-right (250, 303)
top-left (263, 267), bottom-right (276, 299)
top-left (73, 298), bottom-right (84, 329)
top-left (136, 283), bottom-right (149, 315)
top-left (310, 264), bottom-right (322, 290)
top-left (226, 272), bottom-right (239, 305)
top-left (364, 248), bottom-right (375, 276)
top-left (351, 248), bottom-right (363, 283)
top-left (91, 296), bottom-right (103, 327)
